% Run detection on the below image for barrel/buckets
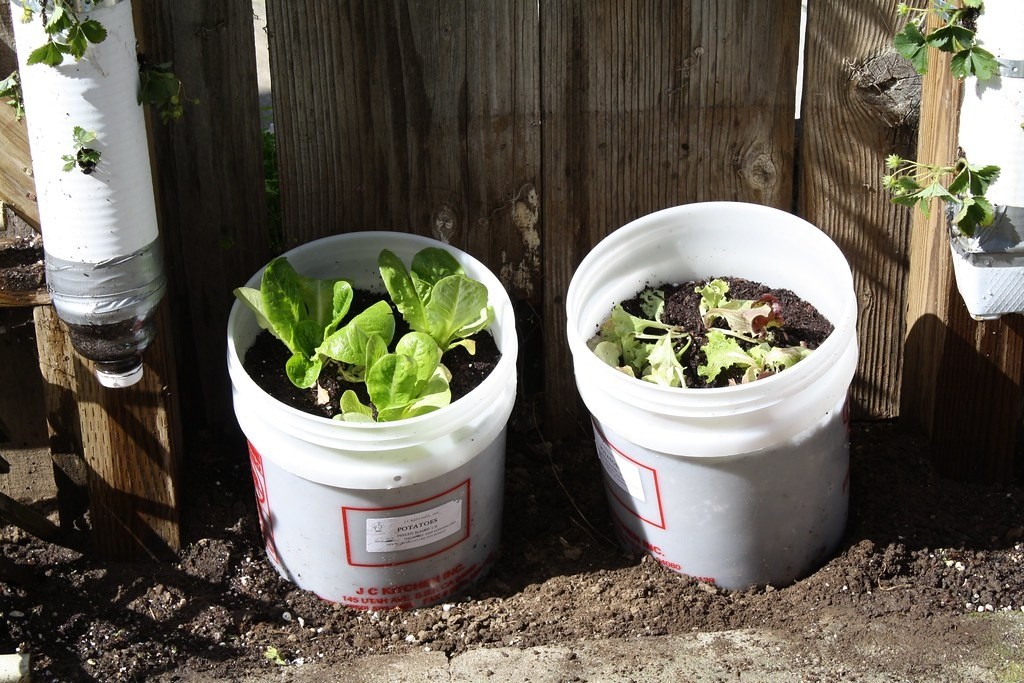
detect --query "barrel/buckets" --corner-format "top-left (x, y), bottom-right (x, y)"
top-left (226, 232), bottom-right (519, 613)
top-left (564, 200), bottom-right (859, 593)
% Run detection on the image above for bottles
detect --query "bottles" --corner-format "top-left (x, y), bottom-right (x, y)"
top-left (60, 313), bottom-right (159, 388)
